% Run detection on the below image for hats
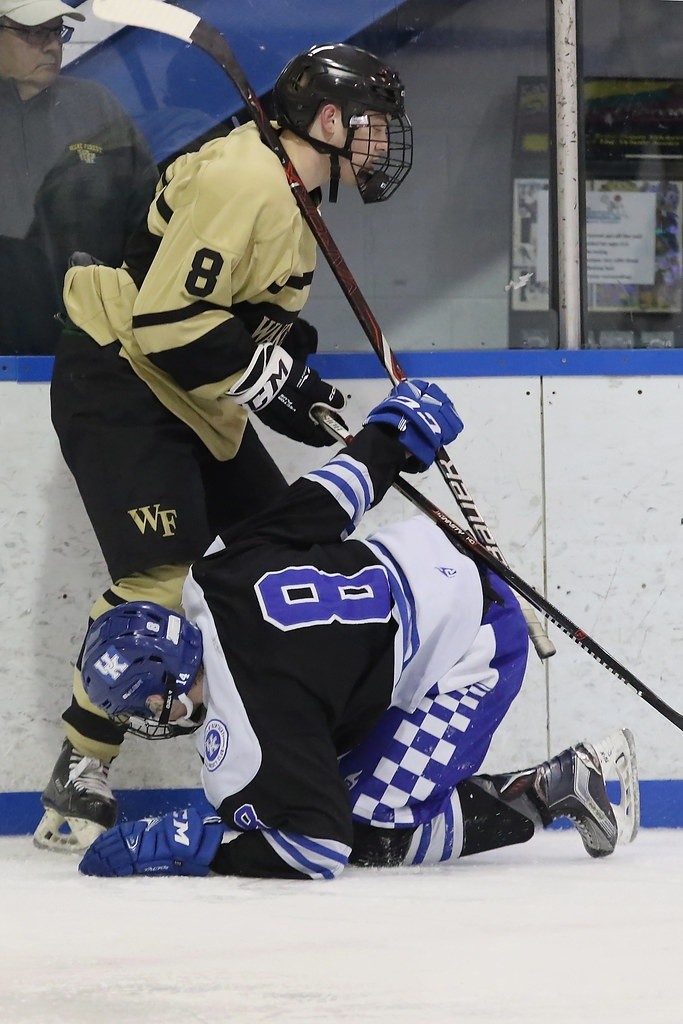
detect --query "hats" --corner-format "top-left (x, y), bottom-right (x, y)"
top-left (0, 0), bottom-right (86, 26)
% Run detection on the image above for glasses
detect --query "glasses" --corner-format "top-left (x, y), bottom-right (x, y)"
top-left (0, 24), bottom-right (74, 45)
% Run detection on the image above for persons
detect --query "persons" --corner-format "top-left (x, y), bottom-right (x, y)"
top-left (31, 42), bottom-right (415, 851)
top-left (0, 0), bottom-right (163, 354)
top-left (79, 378), bottom-right (643, 880)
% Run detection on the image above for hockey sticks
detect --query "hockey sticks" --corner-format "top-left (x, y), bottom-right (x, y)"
top-left (311, 408), bottom-right (683, 733)
top-left (87, 0), bottom-right (558, 663)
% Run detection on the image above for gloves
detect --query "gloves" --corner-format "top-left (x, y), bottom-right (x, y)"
top-left (227, 342), bottom-right (349, 447)
top-left (361, 379), bottom-right (465, 474)
top-left (79, 804), bottom-right (225, 877)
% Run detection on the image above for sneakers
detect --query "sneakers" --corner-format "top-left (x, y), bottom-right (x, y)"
top-left (483, 727), bottom-right (640, 859)
top-left (31, 736), bottom-right (119, 854)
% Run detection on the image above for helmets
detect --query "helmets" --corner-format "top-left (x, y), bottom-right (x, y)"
top-left (273, 43), bottom-right (414, 204)
top-left (81, 600), bottom-right (207, 740)
